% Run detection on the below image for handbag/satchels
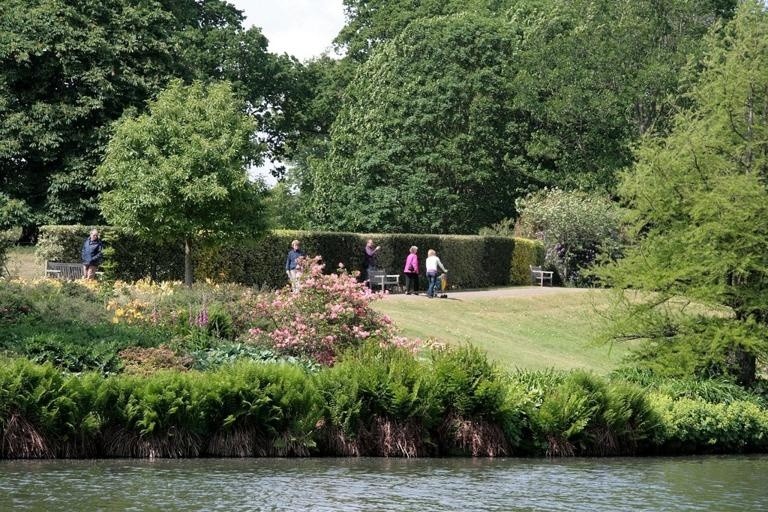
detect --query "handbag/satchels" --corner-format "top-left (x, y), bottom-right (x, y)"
top-left (409, 264), bottom-right (414, 271)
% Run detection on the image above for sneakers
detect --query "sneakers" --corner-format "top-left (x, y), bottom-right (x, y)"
top-left (405, 291), bottom-right (437, 298)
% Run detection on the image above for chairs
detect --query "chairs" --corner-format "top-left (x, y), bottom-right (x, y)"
top-left (366, 268), bottom-right (401, 293)
top-left (529, 264), bottom-right (554, 287)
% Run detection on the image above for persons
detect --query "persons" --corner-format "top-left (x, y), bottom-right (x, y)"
top-left (364, 237), bottom-right (381, 290)
top-left (285, 239), bottom-right (306, 296)
top-left (402, 244), bottom-right (419, 297)
top-left (424, 248), bottom-right (448, 299)
top-left (81, 227), bottom-right (104, 282)
